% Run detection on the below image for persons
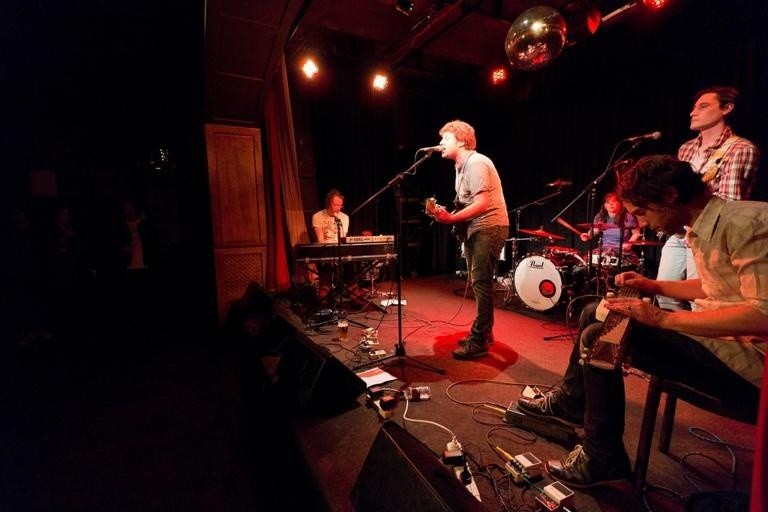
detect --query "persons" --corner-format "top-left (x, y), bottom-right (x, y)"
top-left (516, 156), bottom-right (768, 488)
top-left (579, 193), bottom-right (641, 251)
top-left (654, 87), bottom-right (764, 310)
top-left (426, 120), bottom-right (511, 359)
top-left (312, 188), bottom-right (358, 297)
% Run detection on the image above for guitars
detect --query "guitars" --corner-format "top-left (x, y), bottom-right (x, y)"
top-left (427, 197), bottom-right (468, 258)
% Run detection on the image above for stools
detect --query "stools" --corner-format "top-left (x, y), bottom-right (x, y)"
top-left (623, 370), bottom-right (765, 510)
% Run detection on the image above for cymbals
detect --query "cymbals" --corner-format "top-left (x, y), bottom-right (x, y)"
top-left (518, 229), bottom-right (566, 241)
top-left (623, 240), bottom-right (664, 246)
top-left (577, 223), bottom-right (621, 229)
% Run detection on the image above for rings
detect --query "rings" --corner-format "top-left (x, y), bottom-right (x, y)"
top-left (623, 304), bottom-right (632, 315)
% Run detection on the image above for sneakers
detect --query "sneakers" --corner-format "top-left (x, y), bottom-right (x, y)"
top-left (452, 339), bottom-right (490, 360)
top-left (457, 333), bottom-right (495, 345)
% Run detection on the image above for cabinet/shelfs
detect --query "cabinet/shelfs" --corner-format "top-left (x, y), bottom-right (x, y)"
top-left (382, 182), bottom-right (439, 280)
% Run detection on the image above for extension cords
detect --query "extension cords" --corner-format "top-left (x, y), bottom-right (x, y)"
top-left (450, 458), bottom-right (484, 502)
top-left (366, 385), bottom-right (396, 421)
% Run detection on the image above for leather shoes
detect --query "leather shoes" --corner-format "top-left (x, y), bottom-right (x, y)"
top-left (543, 444), bottom-right (633, 489)
top-left (517, 388), bottom-right (585, 428)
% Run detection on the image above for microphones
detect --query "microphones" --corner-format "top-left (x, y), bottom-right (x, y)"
top-left (629, 129), bottom-right (665, 143)
top-left (419, 142), bottom-right (448, 156)
top-left (330, 210), bottom-right (343, 228)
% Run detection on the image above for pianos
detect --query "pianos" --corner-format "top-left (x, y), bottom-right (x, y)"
top-left (294, 242), bottom-right (398, 263)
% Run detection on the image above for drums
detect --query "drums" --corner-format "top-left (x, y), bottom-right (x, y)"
top-left (592, 253), bottom-right (634, 268)
top-left (544, 245), bottom-right (580, 253)
top-left (513, 252), bottom-right (589, 312)
